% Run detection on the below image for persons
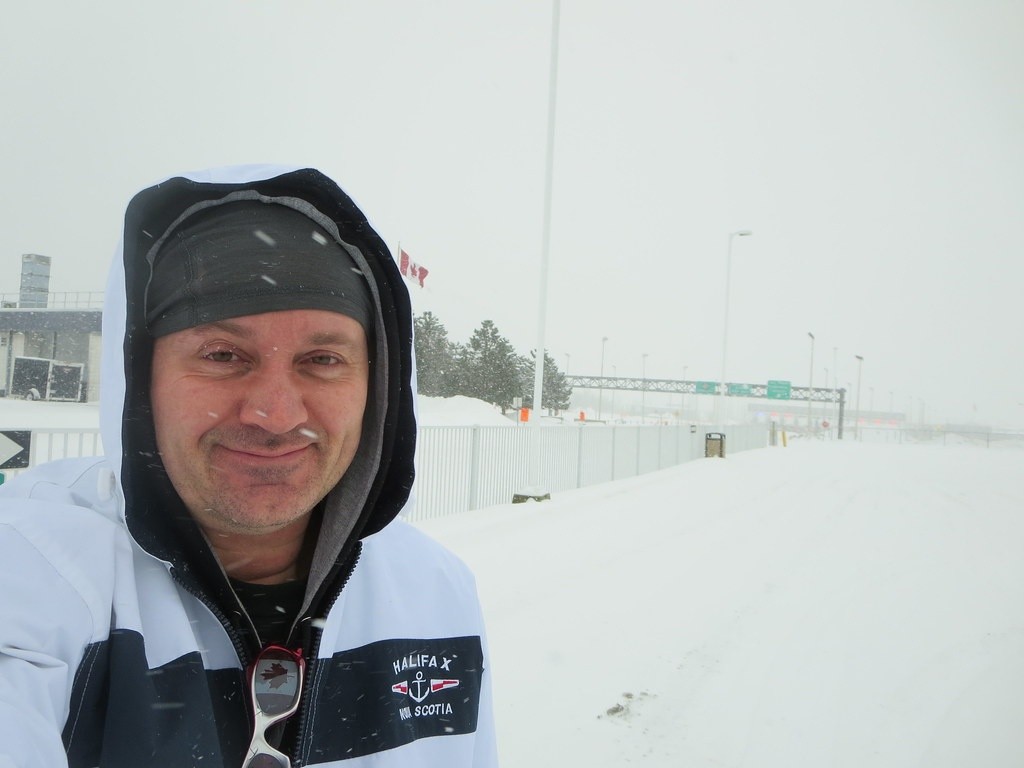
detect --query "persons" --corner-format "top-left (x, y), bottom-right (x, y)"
top-left (0, 163), bottom-right (499, 768)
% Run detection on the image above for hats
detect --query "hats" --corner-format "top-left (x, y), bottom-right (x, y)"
top-left (145, 198), bottom-right (379, 371)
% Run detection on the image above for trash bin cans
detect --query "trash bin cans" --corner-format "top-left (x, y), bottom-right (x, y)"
top-left (705, 432), bottom-right (726, 459)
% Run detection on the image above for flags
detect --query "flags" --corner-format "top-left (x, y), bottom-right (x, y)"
top-left (399, 248), bottom-right (429, 288)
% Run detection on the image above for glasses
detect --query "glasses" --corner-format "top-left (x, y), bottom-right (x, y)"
top-left (241, 645), bottom-right (306, 768)
